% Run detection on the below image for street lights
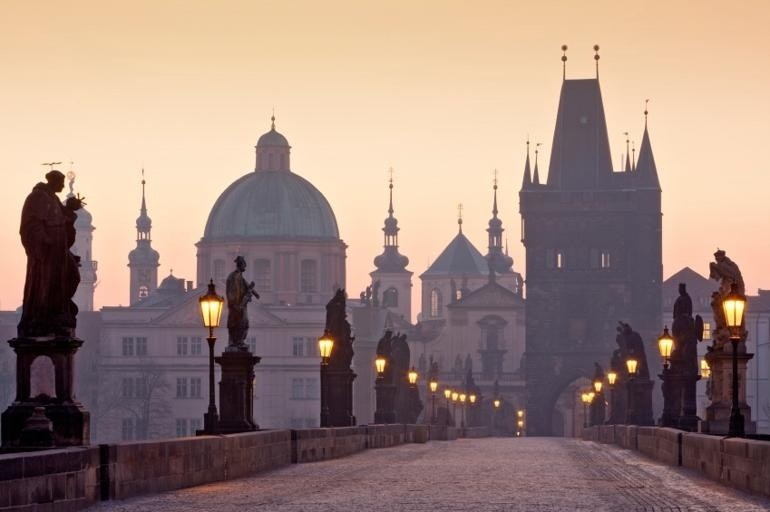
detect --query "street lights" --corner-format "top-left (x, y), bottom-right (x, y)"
top-left (607, 370), bottom-right (615, 412)
top-left (594, 380), bottom-right (603, 424)
top-left (657, 324), bottom-right (674, 395)
top-left (373, 351), bottom-right (387, 422)
top-left (517, 408), bottom-right (524, 437)
top-left (430, 378), bottom-right (477, 430)
top-left (408, 365), bottom-right (419, 423)
top-left (316, 326), bottom-right (336, 428)
top-left (625, 357), bottom-right (639, 383)
top-left (196, 276), bottom-right (226, 434)
top-left (580, 391), bottom-right (589, 428)
top-left (719, 274), bottom-right (753, 438)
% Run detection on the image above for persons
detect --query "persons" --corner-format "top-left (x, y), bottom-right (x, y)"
top-left (592, 320), bottom-right (650, 394)
top-left (672, 282), bottom-right (704, 373)
top-left (374, 329), bottom-right (474, 384)
top-left (17, 170), bottom-right (84, 336)
top-left (708, 247), bottom-right (746, 351)
top-left (225, 254), bottom-right (261, 347)
top-left (324, 287), bottom-right (356, 372)
